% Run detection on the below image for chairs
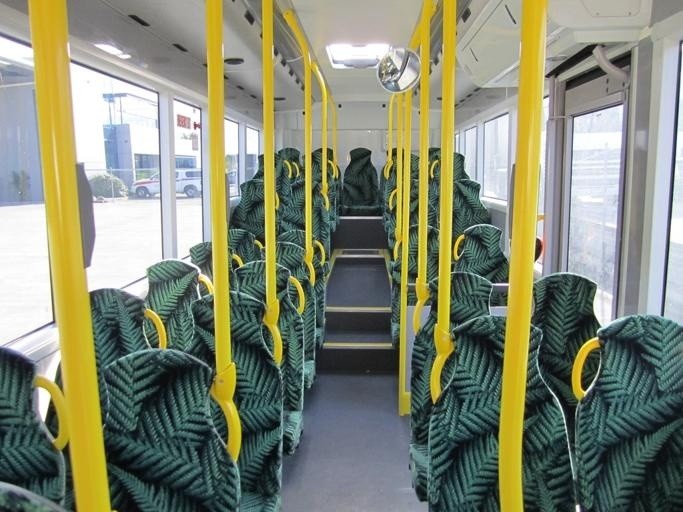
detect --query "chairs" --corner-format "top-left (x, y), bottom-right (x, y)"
top-left (340, 146), bottom-right (381, 213)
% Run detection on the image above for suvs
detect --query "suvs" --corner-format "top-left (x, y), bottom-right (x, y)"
top-left (130, 168), bottom-right (202, 197)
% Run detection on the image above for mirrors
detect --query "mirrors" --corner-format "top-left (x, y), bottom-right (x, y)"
top-left (377, 47), bottom-right (422, 92)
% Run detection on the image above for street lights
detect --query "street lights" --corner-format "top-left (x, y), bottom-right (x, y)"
top-left (102, 93), bottom-right (133, 186)
